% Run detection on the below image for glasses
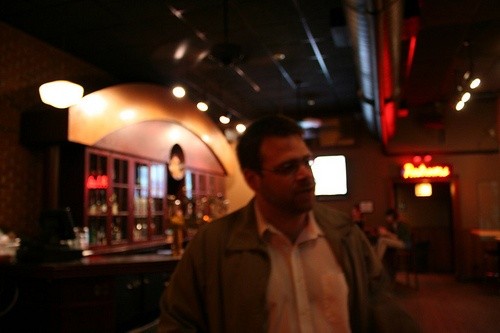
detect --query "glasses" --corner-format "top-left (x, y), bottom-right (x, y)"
top-left (261, 157), bottom-right (315, 175)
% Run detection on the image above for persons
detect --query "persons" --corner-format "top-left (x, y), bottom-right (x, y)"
top-left (376, 208), bottom-right (418, 291)
top-left (158, 116), bottom-right (421, 333)
top-left (352, 220), bottom-right (414, 264)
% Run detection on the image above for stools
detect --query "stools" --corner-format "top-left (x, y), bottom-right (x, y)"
top-left (389, 249), bottom-right (418, 289)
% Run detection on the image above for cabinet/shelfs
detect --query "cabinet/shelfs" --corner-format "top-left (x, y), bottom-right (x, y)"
top-left (59, 146), bottom-right (225, 247)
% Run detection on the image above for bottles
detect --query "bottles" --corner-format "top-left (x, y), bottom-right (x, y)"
top-left (85, 161), bottom-right (228, 256)
